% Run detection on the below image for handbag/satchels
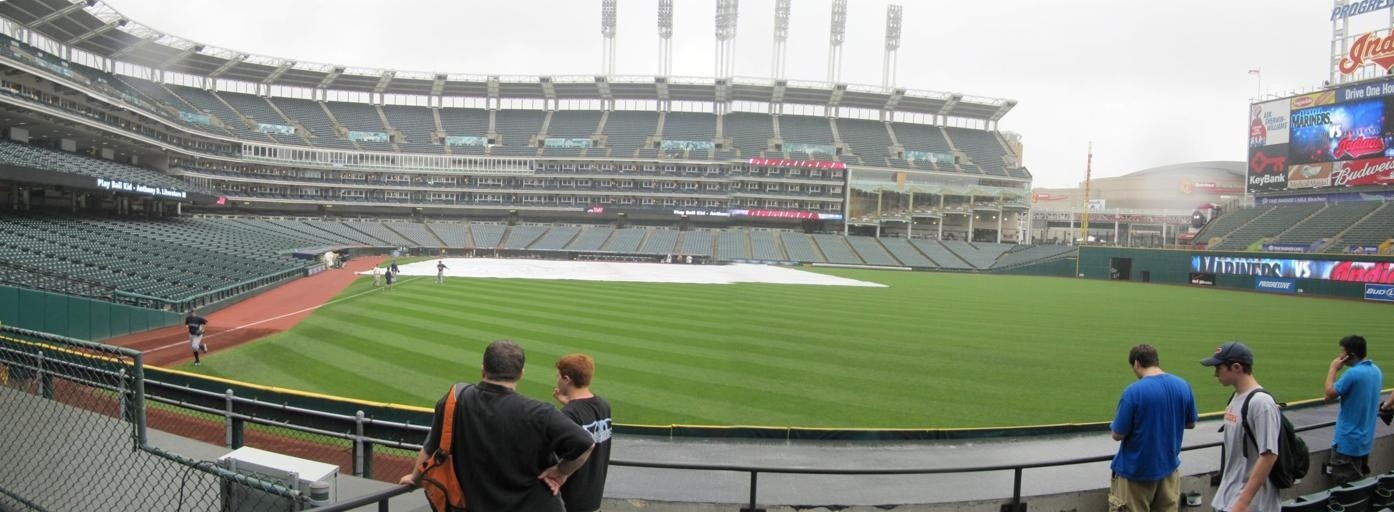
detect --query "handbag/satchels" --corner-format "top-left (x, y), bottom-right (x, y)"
top-left (423, 448), bottom-right (467, 512)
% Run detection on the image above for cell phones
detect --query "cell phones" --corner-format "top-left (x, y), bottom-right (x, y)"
top-left (1341, 352), bottom-right (1354, 363)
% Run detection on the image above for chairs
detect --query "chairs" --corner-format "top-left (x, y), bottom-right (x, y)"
top-left (1195, 200), bottom-right (1394, 252)
top-left (1280, 470), bottom-right (1394, 512)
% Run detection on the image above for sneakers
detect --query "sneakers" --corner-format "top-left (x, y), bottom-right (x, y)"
top-left (203, 344), bottom-right (207, 353)
top-left (194, 361), bottom-right (200, 366)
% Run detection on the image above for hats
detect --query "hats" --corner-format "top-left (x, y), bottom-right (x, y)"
top-left (1199, 342), bottom-right (1253, 366)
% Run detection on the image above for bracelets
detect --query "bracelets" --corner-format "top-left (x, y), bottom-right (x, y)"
top-left (1238, 497), bottom-right (1250, 506)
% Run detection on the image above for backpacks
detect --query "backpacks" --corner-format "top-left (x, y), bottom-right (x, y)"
top-left (1241, 389), bottom-right (1311, 489)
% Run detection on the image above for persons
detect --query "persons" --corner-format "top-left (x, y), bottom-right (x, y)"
top-left (372, 260), bottom-right (400, 289)
top-left (1324, 336), bottom-right (1383, 487)
top-left (437, 261), bottom-right (449, 283)
top-left (399, 340), bottom-right (596, 512)
top-left (1107, 344), bottom-right (1199, 512)
top-left (1382, 391), bottom-right (1394, 410)
top-left (552, 353), bottom-right (613, 512)
top-left (1200, 340), bottom-right (1282, 512)
top-left (185, 308), bottom-right (208, 366)
top-left (343, 254), bottom-right (347, 268)
